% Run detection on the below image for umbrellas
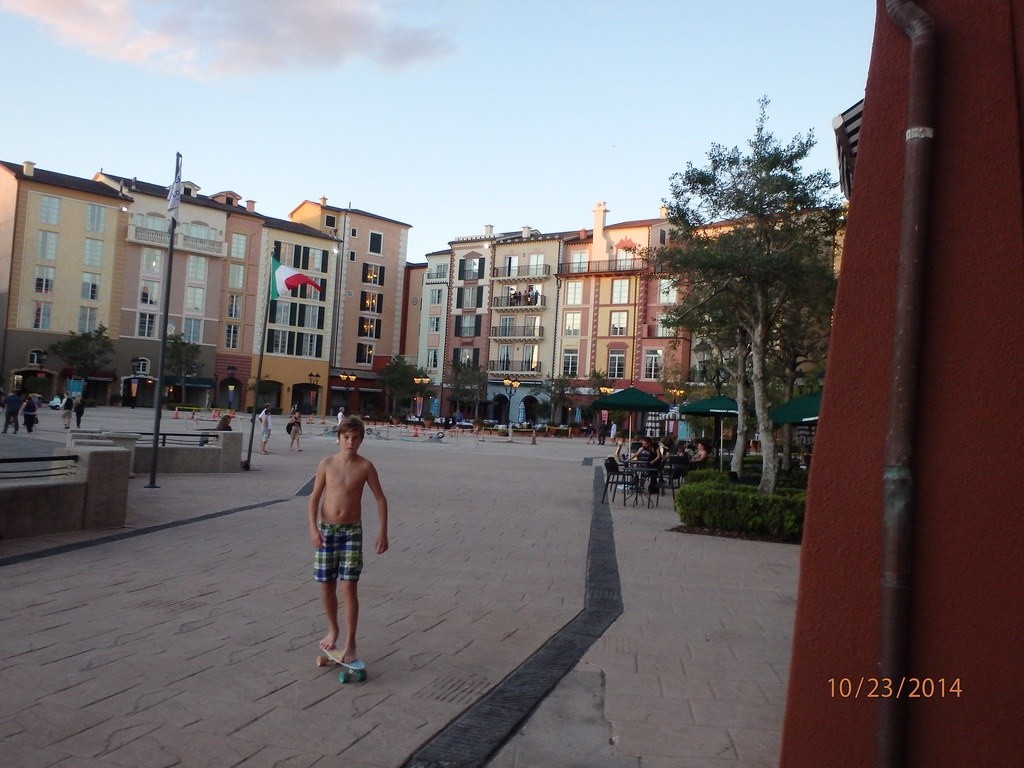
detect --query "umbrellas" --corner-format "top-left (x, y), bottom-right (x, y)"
top-left (680, 396), bottom-right (752, 473)
top-left (592, 387), bottom-right (669, 472)
top-left (771, 390), bottom-right (822, 444)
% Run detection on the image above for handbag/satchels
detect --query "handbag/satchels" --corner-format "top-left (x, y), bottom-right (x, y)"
top-left (286, 423), bottom-right (294, 434)
top-left (605, 457), bottom-right (619, 472)
top-left (33, 417), bottom-right (39, 424)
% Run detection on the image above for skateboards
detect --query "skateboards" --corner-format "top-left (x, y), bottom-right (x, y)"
top-left (317, 646), bottom-right (367, 684)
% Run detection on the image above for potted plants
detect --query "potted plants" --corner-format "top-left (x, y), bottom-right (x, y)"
top-left (423, 412), bottom-right (435, 429)
top-left (390, 412), bottom-right (402, 427)
top-left (472, 418), bottom-right (483, 435)
top-left (498, 429), bottom-right (508, 436)
top-left (568, 421), bottom-right (583, 436)
top-left (548, 422), bottom-right (557, 435)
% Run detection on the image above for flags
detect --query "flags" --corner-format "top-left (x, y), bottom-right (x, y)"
top-left (271, 256), bottom-right (321, 300)
top-left (166, 154), bottom-right (182, 233)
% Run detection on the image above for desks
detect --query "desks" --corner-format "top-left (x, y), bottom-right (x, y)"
top-left (624, 467), bottom-right (661, 509)
top-left (629, 460), bottom-right (649, 466)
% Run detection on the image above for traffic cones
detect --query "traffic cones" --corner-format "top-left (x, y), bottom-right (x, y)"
top-left (210, 410), bottom-right (216, 421)
top-left (216, 409), bottom-right (221, 417)
top-left (413, 428), bottom-right (419, 437)
top-left (530, 431), bottom-right (537, 445)
top-left (173, 408), bottom-right (180, 419)
top-left (228, 408), bottom-right (235, 418)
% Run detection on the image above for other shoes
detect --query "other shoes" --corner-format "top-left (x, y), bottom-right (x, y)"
top-left (598, 443), bottom-right (601, 445)
top-left (262, 452), bottom-right (269, 455)
top-left (593, 441), bottom-right (595, 444)
top-left (602, 443), bottom-right (604, 445)
top-left (297, 449), bottom-right (302, 452)
top-left (65, 426), bottom-right (69, 429)
top-left (2, 431), bottom-right (7, 433)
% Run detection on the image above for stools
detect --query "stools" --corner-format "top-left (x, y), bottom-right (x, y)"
top-left (600, 444), bottom-right (708, 511)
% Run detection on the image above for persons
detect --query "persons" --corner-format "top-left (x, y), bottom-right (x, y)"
top-left (259, 403), bottom-right (270, 455)
top-left (61, 392), bottom-right (84, 429)
top-left (629, 437), bottom-right (661, 465)
top-left (598, 421), bottom-right (607, 445)
top-left (289, 402), bottom-right (303, 451)
top-left (674, 440), bottom-right (709, 461)
top-left (336, 407), bottom-right (345, 444)
top-left (308, 416), bottom-right (389, 663)
top-left (512, 288), bottom-right (536, 306)
top-left (611, 421), bottom-right (617, 438)
top-left (216, 409), bottom-right (235, 431)
top-left (0, 389), bottom-right (38, 434)
top-left (456, 410), bottom-right (463, 422)
top-left (584, 422), bottom-right (595, 444)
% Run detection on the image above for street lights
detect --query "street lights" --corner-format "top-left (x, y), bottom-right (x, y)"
top-left (693, 338), bottom-right (738, 452)
top-left (795, 370), bottom-right (804, 397)
top-left (340, 371), bottom-right (357, 407)
top-left (226, 366), bottom-right (236, 408)
top-left (414, 373), bottom-right (431, 422)
top-left (309, 373), bottom-right (320, 416)
top-left (599, 384), bottom-right (615, 425)
top-left (37, 351), bottom-right (47, 392)
top-left (503, 376), bottom-right (521, 428)
top-left (131, 357), bottom-right (140, 407)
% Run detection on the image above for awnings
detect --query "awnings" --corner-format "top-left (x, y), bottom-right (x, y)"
top-left (164, 375), bottom-right (213, 387)
top-left (63, 367), bottom-right (118, 382)
